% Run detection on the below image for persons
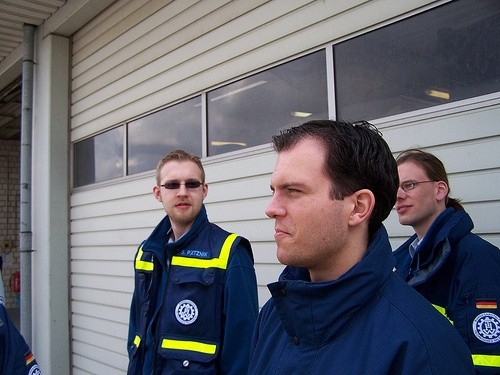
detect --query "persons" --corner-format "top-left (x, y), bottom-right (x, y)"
top-left (124, 149), bottom-right (261, 375)
top-left (386, 145), bottom-right (500, 375)
top-left (246, 118), bottom-right (475, 375)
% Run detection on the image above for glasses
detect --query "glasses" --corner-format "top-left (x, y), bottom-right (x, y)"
top-left (398, 180), bottom-right (438, 192)
top-left (160, 181), bottom-right (203, 189)
